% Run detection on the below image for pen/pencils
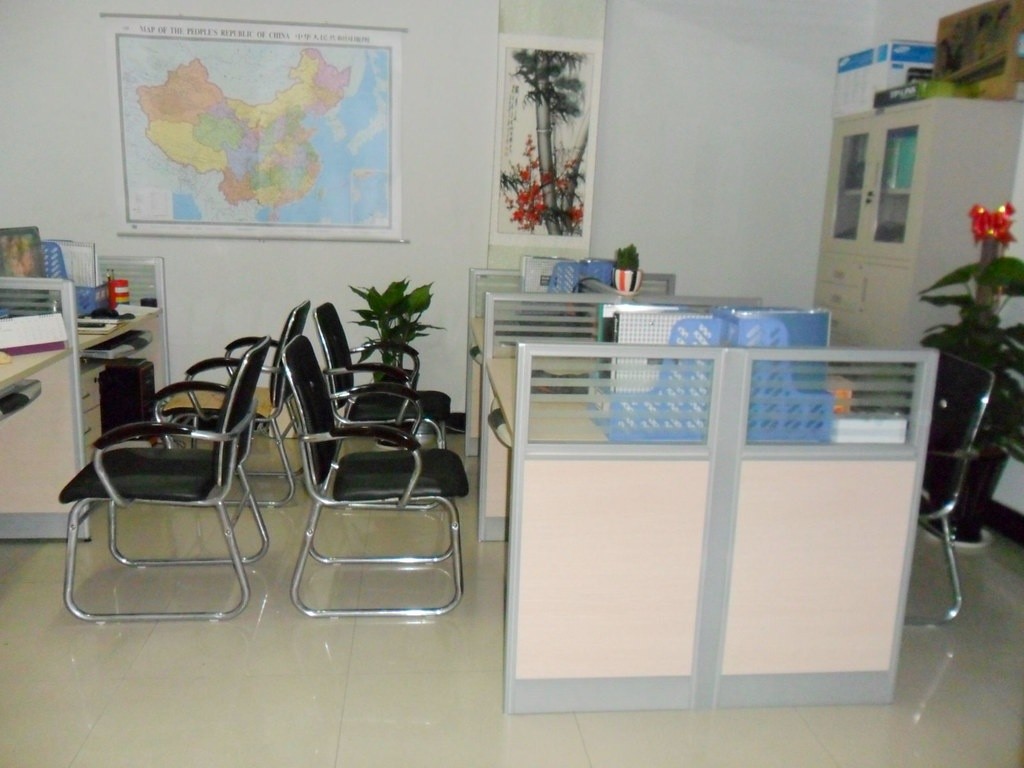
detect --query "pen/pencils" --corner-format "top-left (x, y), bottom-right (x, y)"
top-left (106, 269), bottom-right (114, 281)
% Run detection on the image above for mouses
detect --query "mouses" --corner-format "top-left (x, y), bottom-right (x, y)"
top-left (91, 307), bottom-right (119, 320)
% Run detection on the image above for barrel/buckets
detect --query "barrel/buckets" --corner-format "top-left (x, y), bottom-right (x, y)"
top-left (108, 280), bottom-right (130, 310)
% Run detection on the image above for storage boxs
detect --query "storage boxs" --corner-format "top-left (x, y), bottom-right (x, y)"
top-left (934, 0), bottom-right (1024, 100)
top-left (835, 39), bottom-right (935, 115)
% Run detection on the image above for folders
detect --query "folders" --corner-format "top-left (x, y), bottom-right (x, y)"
top-left (743, 313), bottom-right (828, 393)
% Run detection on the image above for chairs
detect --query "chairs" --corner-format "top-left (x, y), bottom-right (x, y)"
top-left (905, 352), bottom-right (995, 625)
top-left (281, 301), bottom-right (469, 616)
top-left (58, 300), bottom-right (311, 622)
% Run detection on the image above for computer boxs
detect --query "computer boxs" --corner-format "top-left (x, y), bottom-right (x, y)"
top-left (99, 358), bottom-right (156, 434)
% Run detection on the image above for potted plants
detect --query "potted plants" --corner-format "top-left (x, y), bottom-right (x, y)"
top-left (613, 247), bottom-right (643, 291)
top-left (918, 237), bottom-right (1024, 544)
top-left (347, 277), bottom-right (447, 391)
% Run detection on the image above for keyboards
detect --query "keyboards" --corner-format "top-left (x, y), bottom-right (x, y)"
top-left (86, 330), bottom-right (144, 350)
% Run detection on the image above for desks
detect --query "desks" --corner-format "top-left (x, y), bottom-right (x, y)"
top-left (466, 267), bottom-right (940, 711)
top-left (0, 255), bottom-right (171, 542)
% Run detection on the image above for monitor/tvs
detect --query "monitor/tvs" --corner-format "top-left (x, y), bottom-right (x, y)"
top-left (0, 226), bottom-right (51, 317)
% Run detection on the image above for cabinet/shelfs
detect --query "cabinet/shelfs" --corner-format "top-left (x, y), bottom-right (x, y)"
top-left (82, 363), bottom-right (106, 462)
top-left (812, 99), bottom-right (1024, 351)
